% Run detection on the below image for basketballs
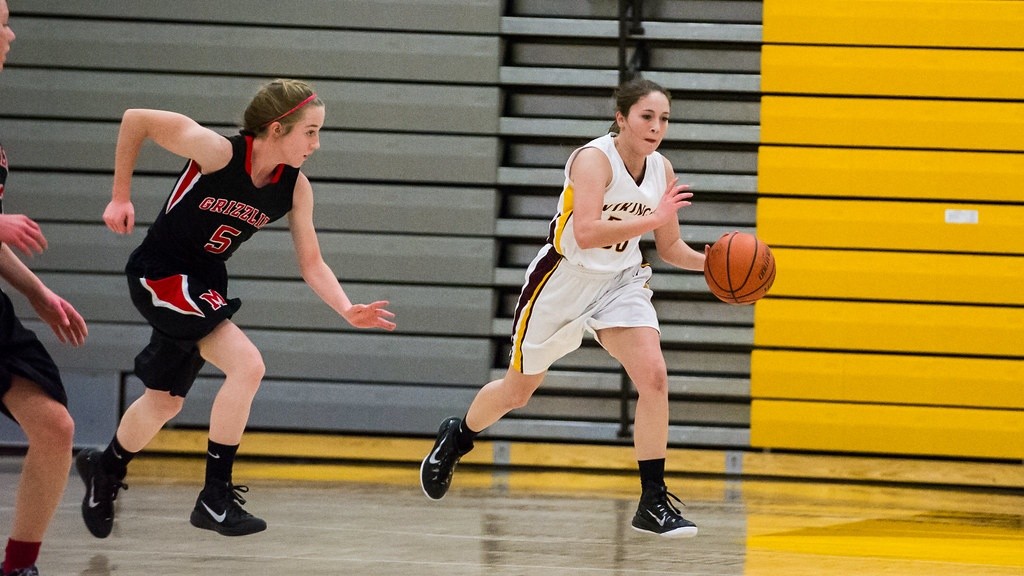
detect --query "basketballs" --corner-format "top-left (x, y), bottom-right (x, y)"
top-left (700, 228), bottom-right (778, 306)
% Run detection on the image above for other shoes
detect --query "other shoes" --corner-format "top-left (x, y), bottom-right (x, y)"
top-left (0, 563), bottom-right (38, 576)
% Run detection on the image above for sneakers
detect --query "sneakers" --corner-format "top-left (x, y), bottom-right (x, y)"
top-left (190, 480), bottom-right (267, 536)
top-left (75, 445), bottom-right (129, 538)
top-left (631, 486), bottom-right (698, 538)
top-left (420, 417), bottom-right (474, 501)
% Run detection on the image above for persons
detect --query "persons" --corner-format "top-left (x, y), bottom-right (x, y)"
top-left (74, 77), bottom-right (396, 539)
top-left (419, 78), bottom-right (741, 540)
top-left (0, 0), bottom-right (88, 575)
